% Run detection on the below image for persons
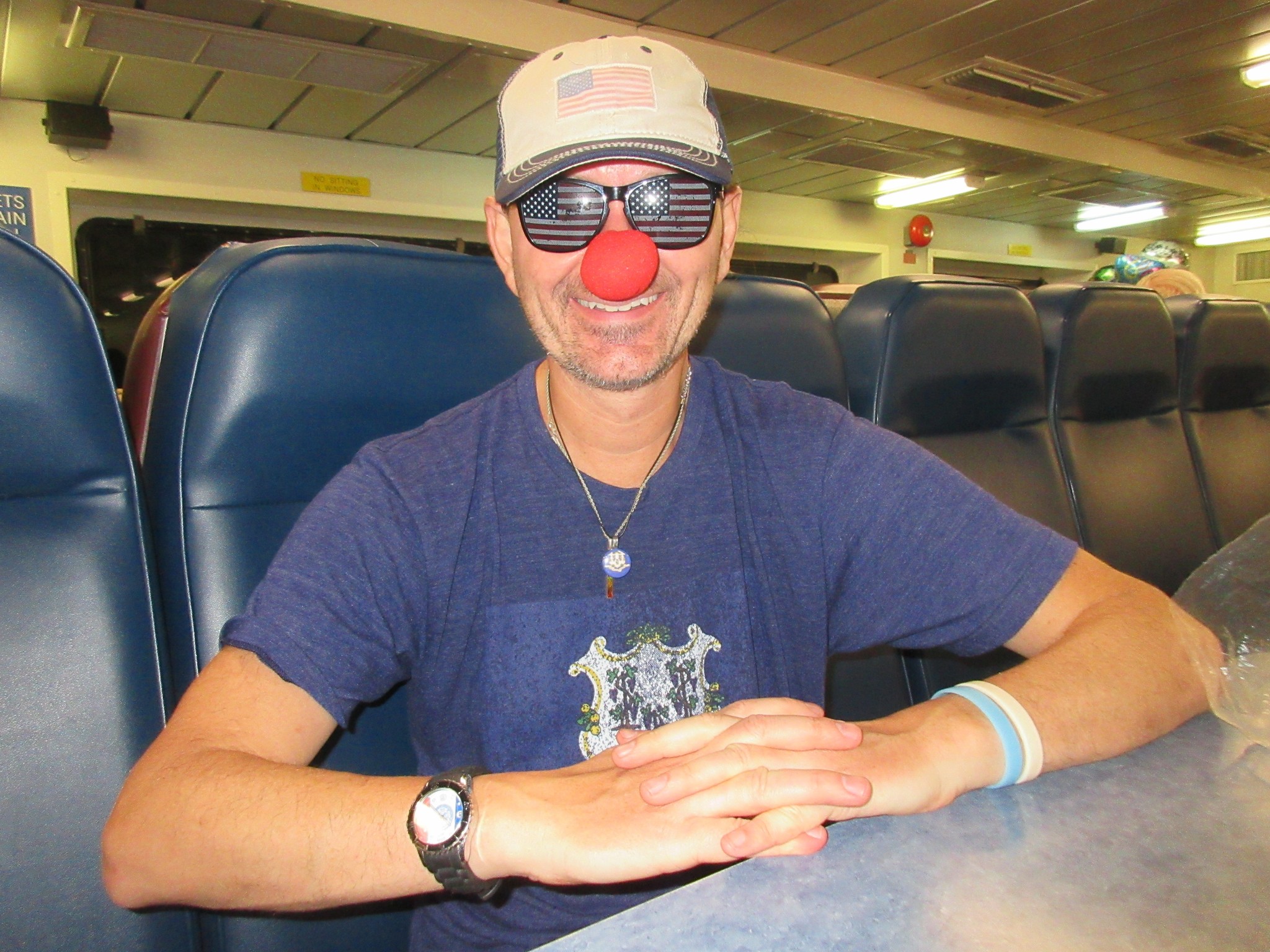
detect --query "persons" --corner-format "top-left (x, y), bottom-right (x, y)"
top-left (96, 34), bottom-right (1227, 910)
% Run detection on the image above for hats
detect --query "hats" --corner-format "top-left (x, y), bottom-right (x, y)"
top-left (495, 35), bottom-right (736, 207)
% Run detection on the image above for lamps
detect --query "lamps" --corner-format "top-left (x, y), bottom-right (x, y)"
top-left (873, 51), bottom-right (1270, 253)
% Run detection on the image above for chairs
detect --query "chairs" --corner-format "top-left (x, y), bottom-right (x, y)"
top-left (0, 217), bottom-right (1270, 952)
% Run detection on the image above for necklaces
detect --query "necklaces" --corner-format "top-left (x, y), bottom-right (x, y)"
top-left (544, 351), bottom-right (694, 579)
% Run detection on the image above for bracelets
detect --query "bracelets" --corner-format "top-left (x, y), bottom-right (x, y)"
top-left (953, 680), bottom-right (1044, 784)
top-left (929, 686), bottom-right (1023, 790)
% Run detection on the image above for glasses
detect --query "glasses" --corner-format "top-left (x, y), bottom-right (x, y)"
top-left (517, 172), bottom-right (727, 254)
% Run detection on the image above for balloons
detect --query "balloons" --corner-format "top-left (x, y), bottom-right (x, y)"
top-left (1085, 238), bottom-right (1193, 286)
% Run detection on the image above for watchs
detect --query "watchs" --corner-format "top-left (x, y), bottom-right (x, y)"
top-left (405, 766), bottom-right (506, 902)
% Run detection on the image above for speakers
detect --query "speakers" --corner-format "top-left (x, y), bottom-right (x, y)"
top-left (40, 99), bottom-right (116, 152)
top-left (1094, 236), bottom-right (1129, 256)
